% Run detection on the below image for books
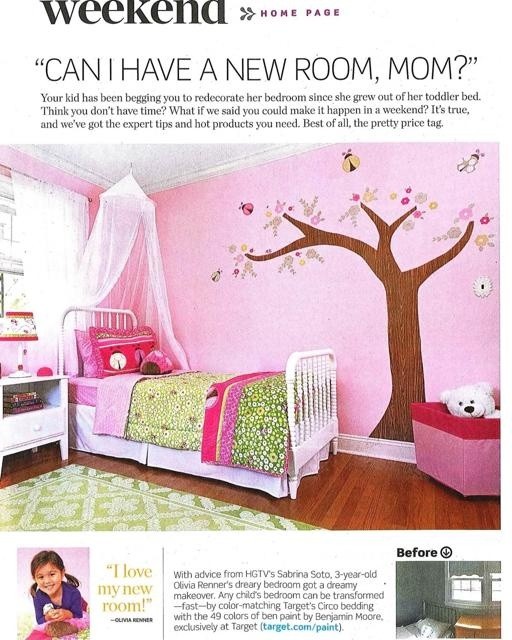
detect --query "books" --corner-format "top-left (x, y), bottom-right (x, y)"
top-left (3, 392), bottom-right (43, 413)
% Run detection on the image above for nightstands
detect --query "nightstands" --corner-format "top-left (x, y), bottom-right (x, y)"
top-left (2, 374), bottom-right (70, 461)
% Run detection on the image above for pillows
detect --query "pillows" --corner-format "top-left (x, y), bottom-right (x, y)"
top-left (73, 323), bottom-right (160, 379)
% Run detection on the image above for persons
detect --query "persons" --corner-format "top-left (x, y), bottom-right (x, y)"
top-left (30, 550), bottom-right (89, 625)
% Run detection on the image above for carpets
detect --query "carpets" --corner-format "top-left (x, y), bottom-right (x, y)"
top-left (0, 462), bottom-right (329, 531)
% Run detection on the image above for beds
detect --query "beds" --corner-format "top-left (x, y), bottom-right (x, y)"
top-left (396, 600), bottom-right (457, 638)
top-left (58, 306), bottom-right (338, 499)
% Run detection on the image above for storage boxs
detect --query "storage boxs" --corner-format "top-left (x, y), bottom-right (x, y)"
top-left (410, 400), bottom-right (501, 495)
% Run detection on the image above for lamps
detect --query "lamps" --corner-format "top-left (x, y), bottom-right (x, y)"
top-left (1, 312), bottom-right (40, 378)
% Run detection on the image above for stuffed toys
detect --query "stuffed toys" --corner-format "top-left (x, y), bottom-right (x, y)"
top-left (440, 381), bottom-right (501, 418)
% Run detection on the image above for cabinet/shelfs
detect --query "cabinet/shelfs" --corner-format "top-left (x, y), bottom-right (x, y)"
top-left (454, 615), bottom-right (501, 638)
